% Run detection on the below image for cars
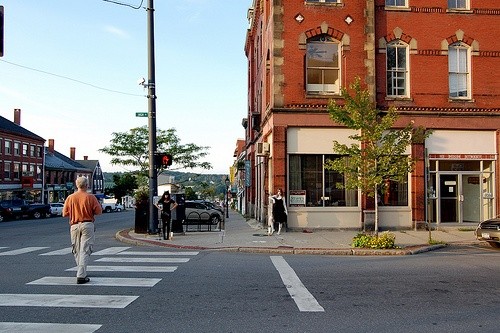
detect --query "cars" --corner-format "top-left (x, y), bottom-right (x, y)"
top-left (474, 217), bottom-right (500, 248)
top-left (0, 199), bottom-right (64, 223)
top-left (101, 201), bottom-right (124, 213)
top-left (185, 200), bottom-right (224, 225)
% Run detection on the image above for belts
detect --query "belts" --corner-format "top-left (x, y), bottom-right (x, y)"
top-left (75, 219), bottom-right (92, 224)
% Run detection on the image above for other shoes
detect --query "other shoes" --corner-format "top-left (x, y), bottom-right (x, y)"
top-left (76, 277), bottom-right (91, 285)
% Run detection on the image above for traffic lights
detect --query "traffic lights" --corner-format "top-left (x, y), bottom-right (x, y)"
top-left (159, 154), bottom-right (172, 166)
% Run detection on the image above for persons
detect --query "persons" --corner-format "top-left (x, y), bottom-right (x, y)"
top-left (62, 176), bottom-right (102, 284)
top-left (154, 191), bottom-right (178, 240)
top-left (268, 187), bottom-right (288, 235)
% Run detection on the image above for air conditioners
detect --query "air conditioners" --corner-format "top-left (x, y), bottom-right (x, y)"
top-left (257, 143), bottom-right (264, 154)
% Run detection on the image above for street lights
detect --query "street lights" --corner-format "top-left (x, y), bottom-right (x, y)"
top-left (225, 175), bottom-right (230, 218)
